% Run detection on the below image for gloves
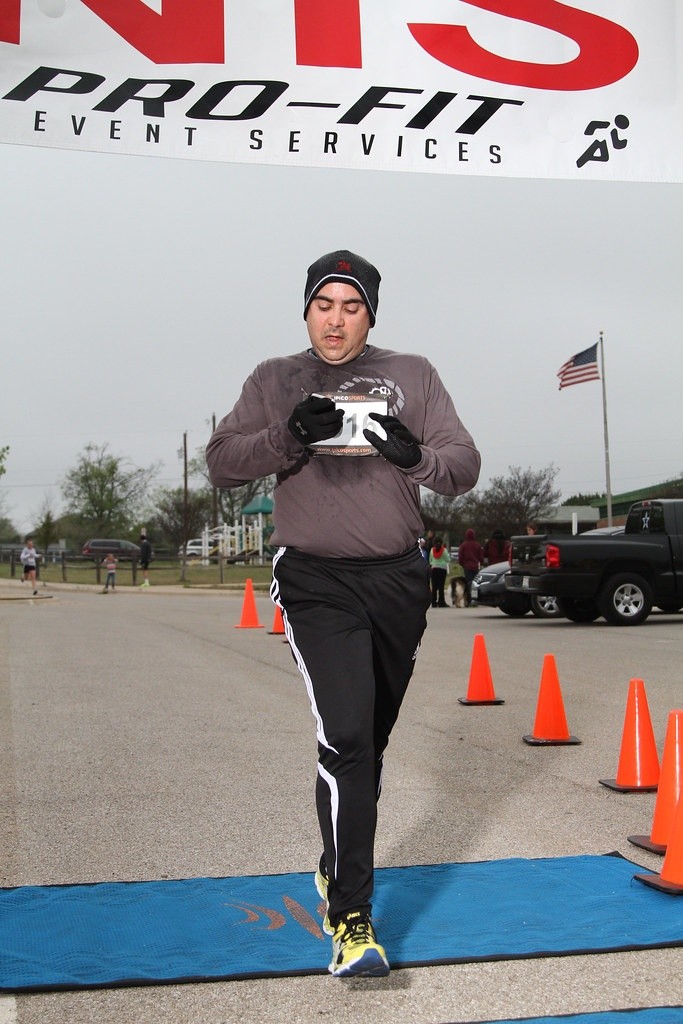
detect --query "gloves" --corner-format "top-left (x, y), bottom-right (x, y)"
top-left (363, 412), bottom-right (421, 469)
top-left (288, 397), bottom-right (344, 446)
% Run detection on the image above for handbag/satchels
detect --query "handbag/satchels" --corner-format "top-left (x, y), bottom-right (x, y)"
top-left (148, 552), bottom-right (156, 563)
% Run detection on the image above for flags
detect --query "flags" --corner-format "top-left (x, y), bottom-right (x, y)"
top-left (556, 341), bottom-right (599, 391)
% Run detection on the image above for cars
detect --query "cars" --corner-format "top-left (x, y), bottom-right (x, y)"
top-left (451, 547), bottom-right (458, 560)
top-left (469, 525), bottom-right (626, 618)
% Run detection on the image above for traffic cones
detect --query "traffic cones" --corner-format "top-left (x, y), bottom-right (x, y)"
top-left (234, 578), bottom-right (265, 628)
top-left (597, 677), bottom-right (661, 792)
top-left (267, 603), bottom-right (287, 634)
top-left (627, 710), bottom-right (683, 898)
top-left (457, 632), bottom-right (505, 706)
top-left (522, 653), bottom-right (582, 746)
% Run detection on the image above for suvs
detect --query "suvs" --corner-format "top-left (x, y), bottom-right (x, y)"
top-left (178, 539), bottom-right (215, 557)
top-left (82, 538), bottom-right (155, 564)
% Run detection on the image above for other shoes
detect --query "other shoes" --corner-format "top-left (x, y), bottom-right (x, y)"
top-left (438, 603), bottom-right (450, 607)
top-left (33, 591), bottom-right (37, 594)
top-left (141, 583), bottom-right (150, 588)
top-left (21, 576), bottom-right (24, 582)
top-left (432, 602), bottom-right (437, 607)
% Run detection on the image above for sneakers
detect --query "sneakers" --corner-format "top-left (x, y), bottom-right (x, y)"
top-left (327, 912), bottom-right (389, 978)
top-left (314, 868), bottom-right (336, 936)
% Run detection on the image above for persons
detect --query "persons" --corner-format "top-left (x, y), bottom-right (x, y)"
top-left (138, 534), bottom-right (151, 587)
top-left (101, 553), bottom-right (118, 593)
top-left (429, 537), bottom-right (450, 608)
top-left (21, 538), bottom-right (43, 595)
top-left (526, 522), bottom-right (538, 537)
top-left (484, 529), bottom-right (511, 567)
top-left (204, 251), bottom-right (481, 977)
top-left (418, 537), bottom-right (428, 570)
top-left (458, 529), bottom-right (484, 607)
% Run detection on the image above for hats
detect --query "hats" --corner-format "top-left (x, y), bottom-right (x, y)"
top-left (303, 250), bottom-right (381, 327)
top-left (465, 529), bottom-right (475, 538)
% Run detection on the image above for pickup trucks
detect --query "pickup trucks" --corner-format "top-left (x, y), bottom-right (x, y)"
top-left (504, 498), bottom-right (683, 626)
